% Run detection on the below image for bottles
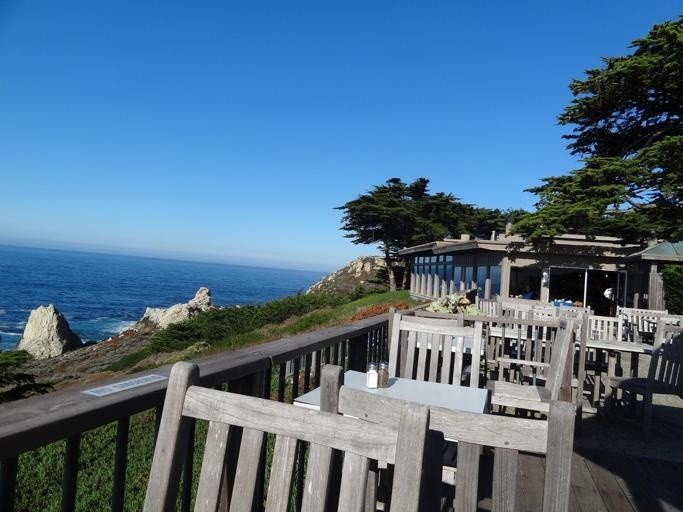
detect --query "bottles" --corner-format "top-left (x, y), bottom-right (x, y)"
top-left (365, 362), bottom-right (377, 388)
top-left (378, 360), bottom-right (389, 388)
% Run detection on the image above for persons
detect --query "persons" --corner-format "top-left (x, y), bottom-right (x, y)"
top-left (521, 285), bottom-right (534, 299)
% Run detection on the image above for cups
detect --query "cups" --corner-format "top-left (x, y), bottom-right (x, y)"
top-left (635, 335), bottom-right (642, 344)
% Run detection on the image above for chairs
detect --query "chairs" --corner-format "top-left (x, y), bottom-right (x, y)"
top-left (142, 295), bottom-right (683, 512)
